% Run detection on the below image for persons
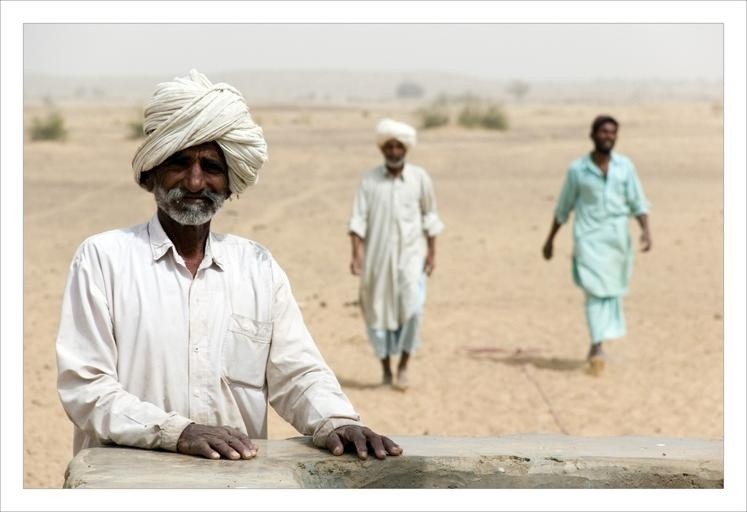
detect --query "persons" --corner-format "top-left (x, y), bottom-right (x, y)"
top-left (50, 69), bottom-right (403, 462)
top-left (540, 114), bottom-right (653, 374)
top-left (347, 117), bottom-right (445, 391)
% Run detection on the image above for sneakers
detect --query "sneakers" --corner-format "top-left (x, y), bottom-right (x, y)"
top-left (586, 353), bottom-right (608, 376)
top-left (383, 373), bottom-right (410, 391)
